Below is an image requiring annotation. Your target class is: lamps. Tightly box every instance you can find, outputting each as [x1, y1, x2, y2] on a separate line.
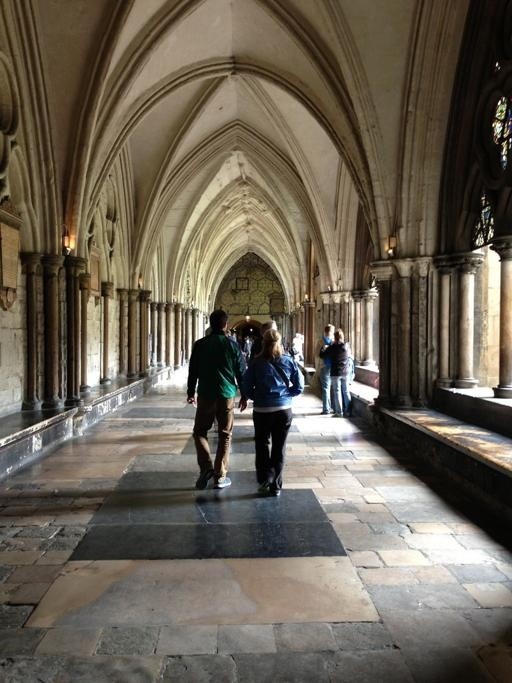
[62, 228, 76, 256]
[388, 235, 398, 250]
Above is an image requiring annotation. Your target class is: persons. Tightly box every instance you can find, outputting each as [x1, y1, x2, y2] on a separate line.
[184, 311, 355, 497]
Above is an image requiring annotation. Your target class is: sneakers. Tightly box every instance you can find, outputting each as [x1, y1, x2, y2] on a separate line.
[215, 477, 231, 488]
[196, 467, 214, 489]
